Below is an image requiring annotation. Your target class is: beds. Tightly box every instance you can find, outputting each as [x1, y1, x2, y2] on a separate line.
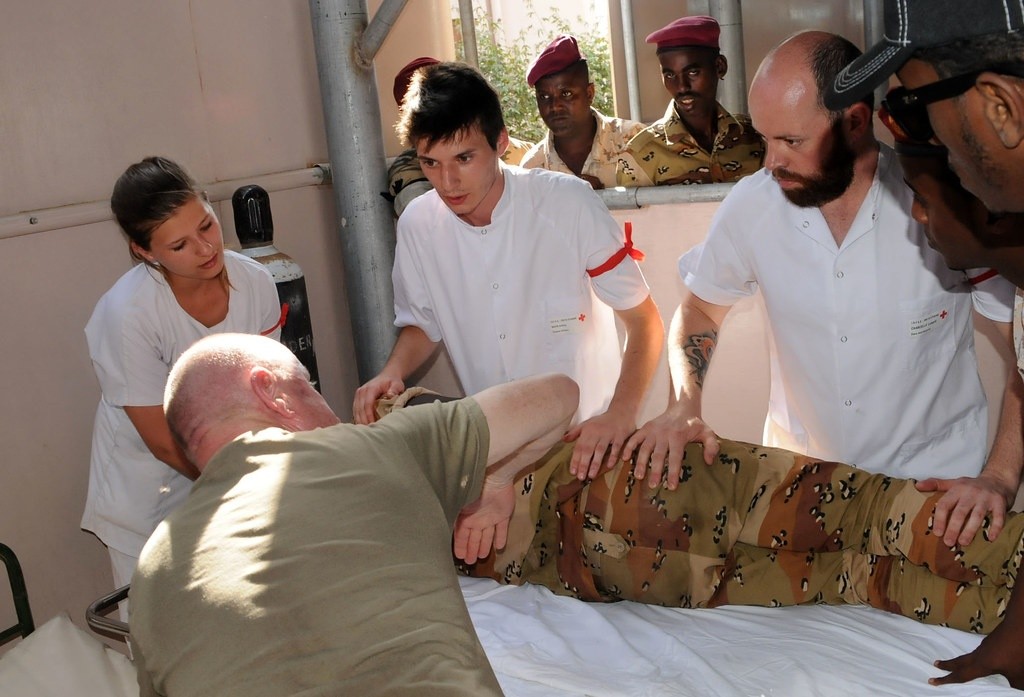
[0, 504, 1024, 697]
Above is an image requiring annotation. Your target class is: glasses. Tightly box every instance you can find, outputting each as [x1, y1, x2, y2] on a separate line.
[880, 64, 1024, 142]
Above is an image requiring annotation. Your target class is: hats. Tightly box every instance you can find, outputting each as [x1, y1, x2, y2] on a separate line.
[526, 34, 581, 88]
[393, 57, 441, 112]
[645, 15, 721, 52]
[823, 0, 1024, 111]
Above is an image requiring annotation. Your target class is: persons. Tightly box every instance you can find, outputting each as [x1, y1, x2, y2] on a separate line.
[448, 430, 1024, 636]
[519, 35, 647, 190]
[79, 157, 281, 623]
[621, 31, 988, 490]
[353, 63, 665, 481]
[822, 0, 1024, 693]
[894, 141, 1024, 547]
[615, 15, 768, 187]
[126, 332, 580, 697]
[388, 56, 536, 216]
